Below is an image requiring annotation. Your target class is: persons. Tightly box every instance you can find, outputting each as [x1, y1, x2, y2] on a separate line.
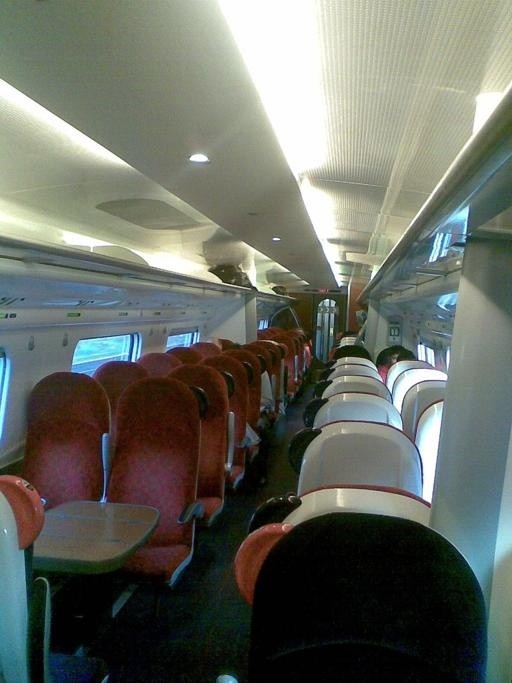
[376, 343, 405, 383]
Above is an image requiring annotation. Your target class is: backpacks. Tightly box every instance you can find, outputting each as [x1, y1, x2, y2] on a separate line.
[208, 263, 257, 290]
